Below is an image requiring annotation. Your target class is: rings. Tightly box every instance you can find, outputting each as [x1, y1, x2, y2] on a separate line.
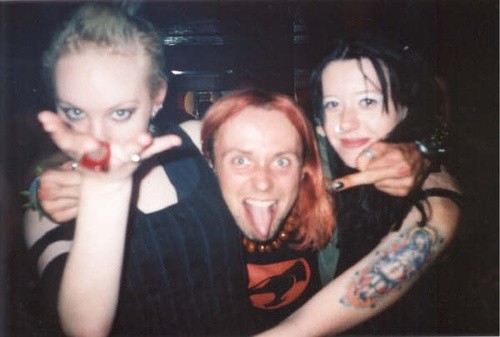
[362, 145, 379, 163]
[129, 153, 142, 165]
[80, 139, 111, 175]
[71, 160, 79, 171]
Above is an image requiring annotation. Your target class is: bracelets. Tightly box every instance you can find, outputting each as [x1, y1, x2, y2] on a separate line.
[21, 161, 64, 223]
[413, 135, 433, 159]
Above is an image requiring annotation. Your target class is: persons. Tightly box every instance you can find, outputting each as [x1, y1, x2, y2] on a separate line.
[250, 38, 474, 336]
[19, 0, 418, 337]
[27, 87, 457, 336]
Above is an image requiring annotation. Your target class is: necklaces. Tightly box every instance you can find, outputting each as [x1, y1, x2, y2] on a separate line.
[242, 200, 309, 256]
